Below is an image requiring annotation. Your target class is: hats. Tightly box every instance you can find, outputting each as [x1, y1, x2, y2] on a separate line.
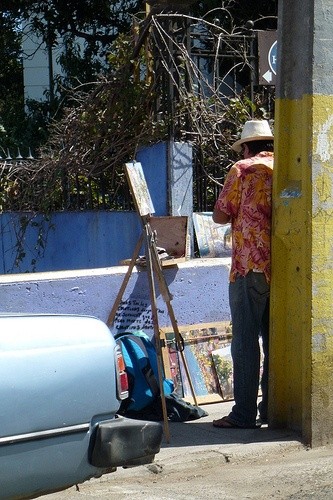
[232, 119, 275, 153]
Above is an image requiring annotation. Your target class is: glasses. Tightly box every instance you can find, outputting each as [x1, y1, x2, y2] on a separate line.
[241, 147, 246, 155]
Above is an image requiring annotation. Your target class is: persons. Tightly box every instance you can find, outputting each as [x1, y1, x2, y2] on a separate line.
[212, 120, 274, 428]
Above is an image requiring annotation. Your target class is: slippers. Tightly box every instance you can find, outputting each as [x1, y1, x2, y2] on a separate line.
[212, 415, 261, 430]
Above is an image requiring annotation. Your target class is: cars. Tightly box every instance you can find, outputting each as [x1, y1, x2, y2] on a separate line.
[0, 308, 165, 500]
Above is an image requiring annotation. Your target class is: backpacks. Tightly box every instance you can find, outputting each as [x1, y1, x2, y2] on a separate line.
[114, 331, 175, 417]
